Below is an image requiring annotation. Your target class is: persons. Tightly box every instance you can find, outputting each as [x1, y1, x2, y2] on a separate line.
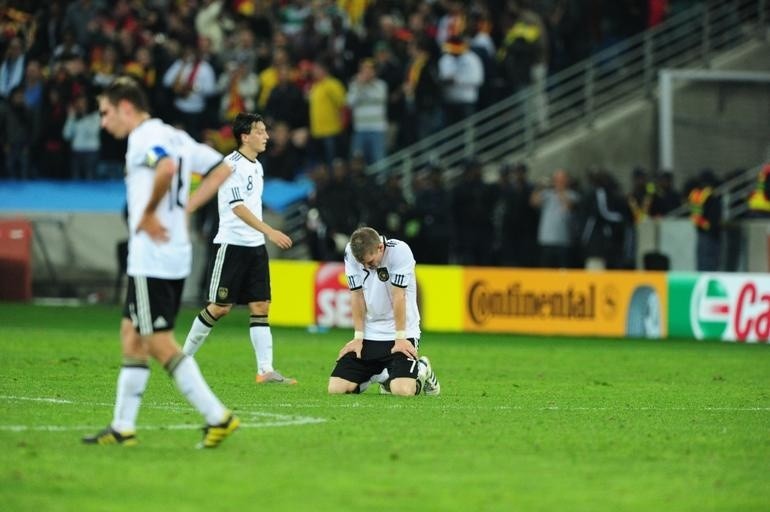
[1, 0, 545, 184]
[303, 154, 750, 272]
[82, 75, 240, 449]
[327, 227, 440, 397]
[538, 0, 749, 78]
[181, 111, 298, 383]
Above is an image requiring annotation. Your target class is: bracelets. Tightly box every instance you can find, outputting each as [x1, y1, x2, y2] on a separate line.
[395, 330, 406, 340]
[354, 330, 364, 339]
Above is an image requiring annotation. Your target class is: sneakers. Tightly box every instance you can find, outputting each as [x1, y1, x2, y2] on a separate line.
[80, 426, 141, 448]
[195, 409, 241, 451]
[378, 376, 391, 395]
[417, 355, 441, 395]
[254, 369, 300, 387]
